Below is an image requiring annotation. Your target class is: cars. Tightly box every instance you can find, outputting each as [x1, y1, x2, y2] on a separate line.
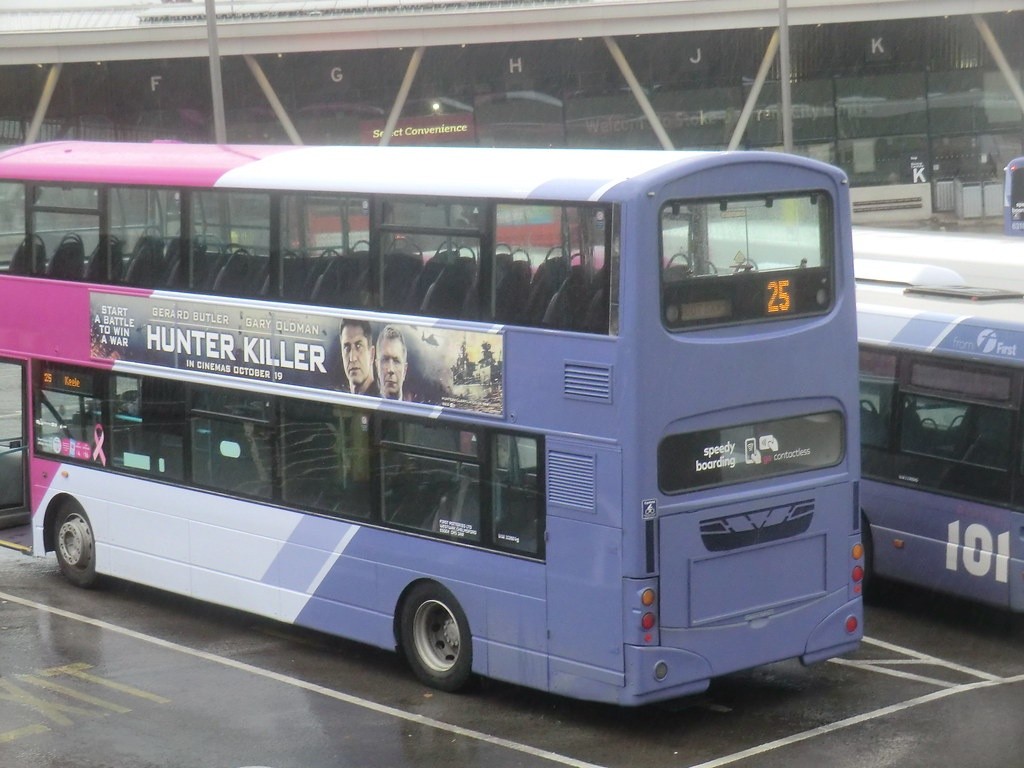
[139, 93, 561, 138]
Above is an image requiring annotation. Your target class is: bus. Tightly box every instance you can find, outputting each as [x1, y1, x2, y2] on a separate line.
[0, 139, 868, 710]
[660, 100, 837, 166]
[1003, 158, 1024, 238]
[830, 90, 989, 176]
[560, 218, 1024, 619]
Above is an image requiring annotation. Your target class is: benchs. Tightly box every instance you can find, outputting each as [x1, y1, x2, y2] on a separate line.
[662, 266, 830, 328]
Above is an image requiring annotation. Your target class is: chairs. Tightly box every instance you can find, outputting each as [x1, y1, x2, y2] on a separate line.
[86, 235, 124, 279]
[9, 234, 47, 276]
[124, 227, 719, 326]
[285, 469, 477, 532]
[860, 400, 964, 430]
[48, 232, 85, 279]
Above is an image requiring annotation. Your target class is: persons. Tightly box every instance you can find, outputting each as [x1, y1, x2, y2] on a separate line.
[367, 324, 432, 404]
[338, 319, 375, 402]
